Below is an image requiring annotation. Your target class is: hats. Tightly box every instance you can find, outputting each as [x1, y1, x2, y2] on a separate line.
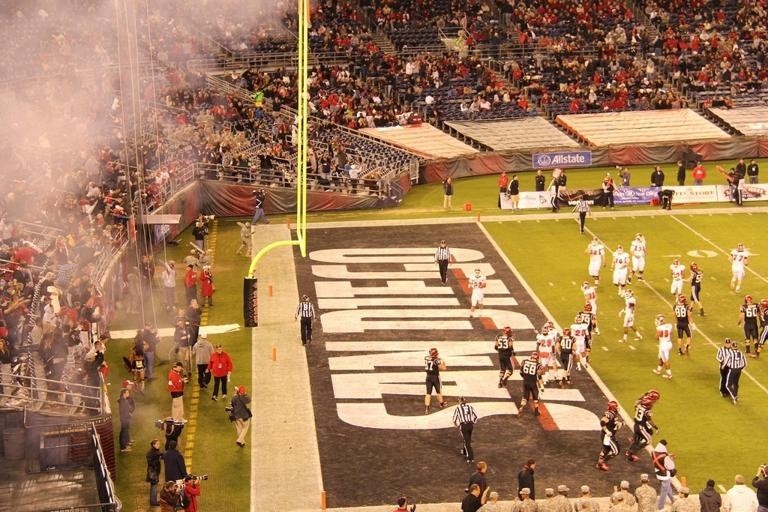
[545, 487, 554, 495]
[677, 486, 690, 494]
[489, 491, 499, 498]
[580, 485, 590, 492]
[614, 492, 623, 500]
[620, 480, 630, 488]
[519, 487, 531, 494]
[558, 484, 570, 491]
[640, 473, 649, 480]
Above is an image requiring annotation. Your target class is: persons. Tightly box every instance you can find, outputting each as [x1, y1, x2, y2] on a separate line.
[294, 294, 315, 345]
[595, 387, 767, 512]
[163, 247, 250, 449]
[572, 200, 591, 233]
[451, 396, 477, 462]
[496, 231, 768, 416]
[145, 418, 207, 512]
[118, 380, 137, 452]
[392, 497, 415, 512]
[0, 187, 159, 410]
[435, 238, 486, 321]
[2, 0, 768, 200]
[423, 348, 449, 415]
[192, 188, 272, 258]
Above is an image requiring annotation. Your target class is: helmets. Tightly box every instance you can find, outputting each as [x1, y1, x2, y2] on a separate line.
[744, 295, 753, 303]
[531, 352, 539, 361]
[641, 398, 652, 409]
[759, 299, 768, 310]
[607, 400, 619, 413]
[542, 282, 592, 336]
[736, 243, 744, 251]
[646, 389, 660, 402]
[590, 233, 699, 325]
[429, 348, 439, 357]
[502, 326, 512, 334]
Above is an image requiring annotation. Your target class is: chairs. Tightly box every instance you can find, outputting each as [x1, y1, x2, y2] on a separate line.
[0, 200, 129, 421]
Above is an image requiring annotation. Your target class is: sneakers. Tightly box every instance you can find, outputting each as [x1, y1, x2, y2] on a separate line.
[597, 461, 608, 470]
[629, 455, 641, 463]
